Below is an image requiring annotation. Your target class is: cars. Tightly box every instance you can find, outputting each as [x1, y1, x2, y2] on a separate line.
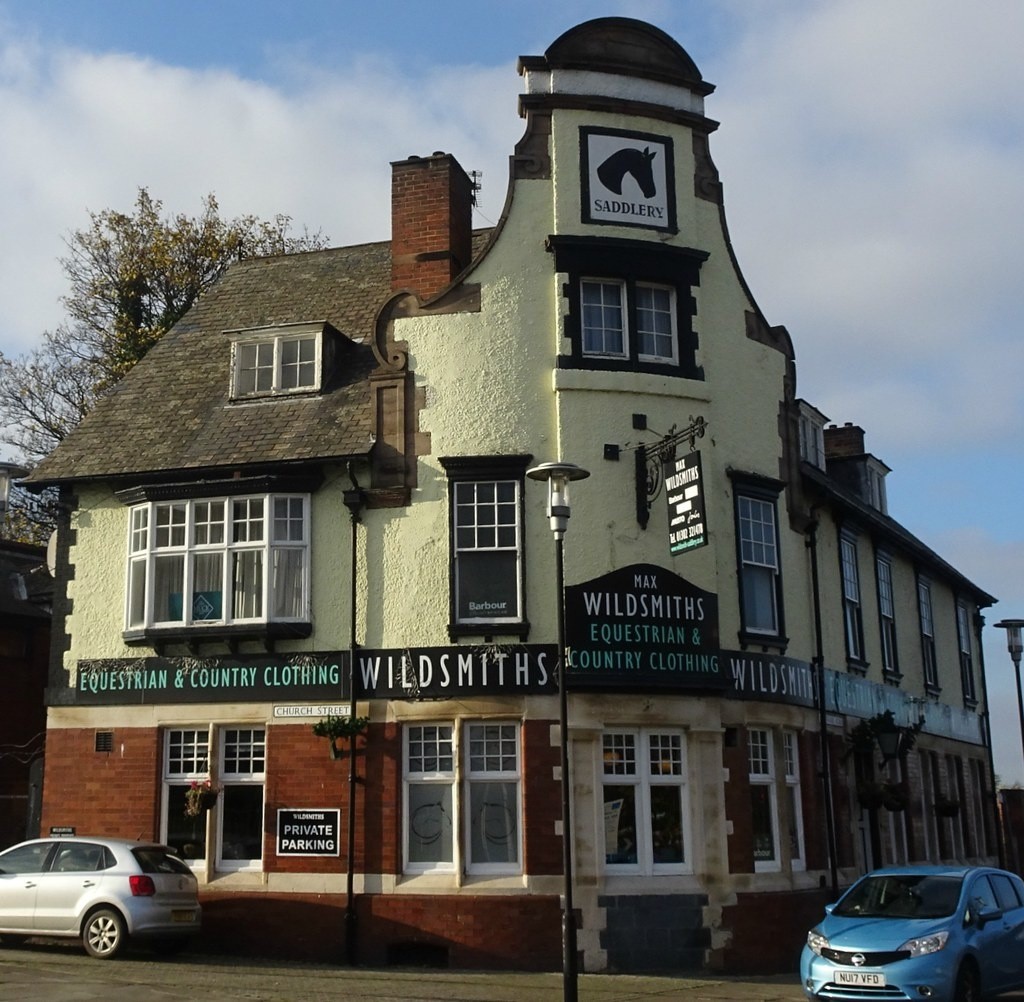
[0, 836, 203, 960]
[799, 865, 1024, 1001]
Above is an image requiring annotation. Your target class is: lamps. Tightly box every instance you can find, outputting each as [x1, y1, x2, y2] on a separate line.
[874, 723, 903, 770]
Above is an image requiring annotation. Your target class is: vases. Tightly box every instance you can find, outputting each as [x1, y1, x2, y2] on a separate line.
[941, 807, 959, 818]
[885, 799, 904, 811]
[199, 792, 215, 809]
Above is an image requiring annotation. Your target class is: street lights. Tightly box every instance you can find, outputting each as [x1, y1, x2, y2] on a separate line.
[526, 462, 591, 1002]
[993, 618, 1024, 751]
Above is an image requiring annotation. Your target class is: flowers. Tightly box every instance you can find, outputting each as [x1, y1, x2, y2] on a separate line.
[876, 781, 912, 802]
[936, 795, 962, 810]
[185, 779, 221, 817]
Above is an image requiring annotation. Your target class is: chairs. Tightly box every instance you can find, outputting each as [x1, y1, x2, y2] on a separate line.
[885, 895, 922, 916]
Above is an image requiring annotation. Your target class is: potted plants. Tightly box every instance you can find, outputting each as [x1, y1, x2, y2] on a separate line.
[311, 717, 371, 762]
